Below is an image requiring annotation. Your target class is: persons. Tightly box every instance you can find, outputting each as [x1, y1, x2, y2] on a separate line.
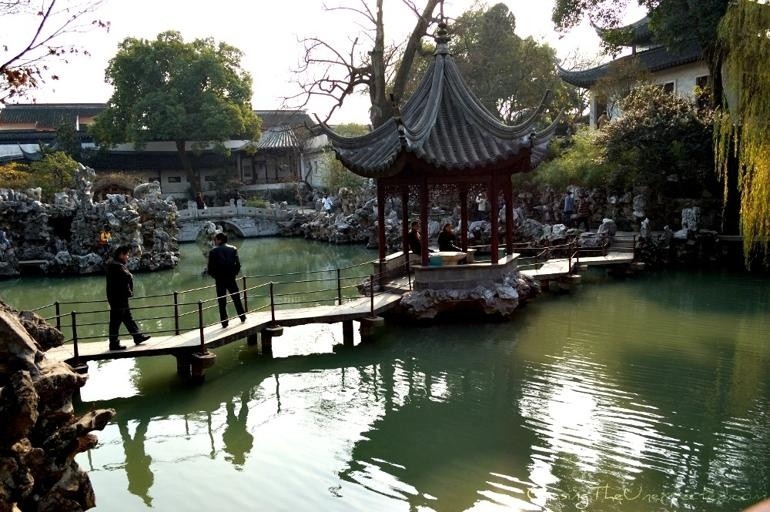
[321, 193, 335, 214]
[233, 188, 241, 206]
[115, 416, 158, 511]
[100, 225, 112, 258]
[207, 232, 247, 328]
[314, 195, 324, 212]
[409, 219, 435, 258]
[104, 245, 151, 351]
[437, 223, 462, 252]
[218, 389, 256, 472]
[467, 185, 593, 233]
[195, 191, 205, 210]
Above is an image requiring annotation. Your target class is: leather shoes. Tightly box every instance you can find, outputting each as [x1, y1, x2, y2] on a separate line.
[133, 335, 151, 345]
[110, 343, 128, 351]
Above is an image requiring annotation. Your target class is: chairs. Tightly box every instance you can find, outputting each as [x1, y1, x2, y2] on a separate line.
[429, 255, 442, 267]
